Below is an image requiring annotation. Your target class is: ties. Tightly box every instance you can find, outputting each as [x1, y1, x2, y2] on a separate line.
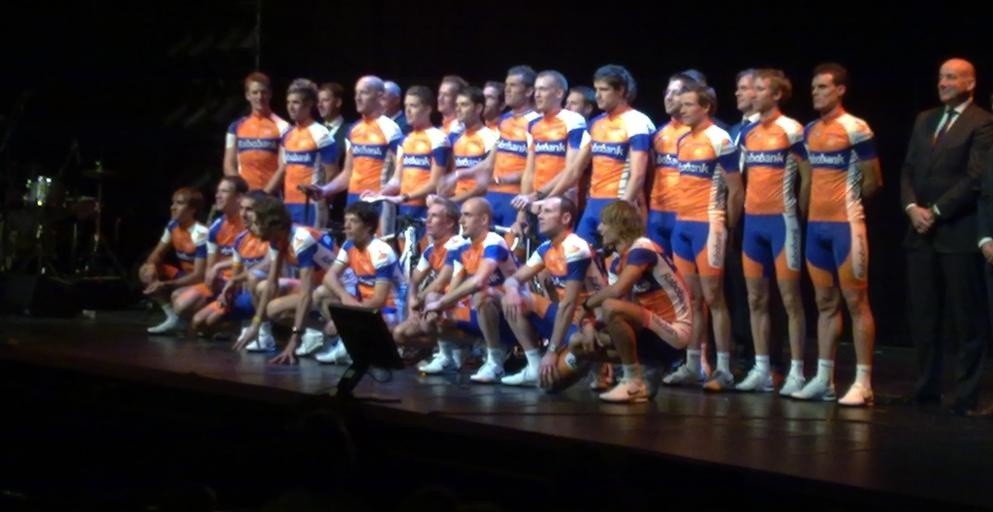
[327, 125, 333, 132]
[935, 110, 956, 143]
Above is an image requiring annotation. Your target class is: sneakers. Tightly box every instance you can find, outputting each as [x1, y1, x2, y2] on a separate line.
[599, 378, 651, 404]
[243, 335, 277, 351]
[418, 355, 459, 375]
[663, 365, 706, 387]
[778, 372, 807, 397]
[790, 378, 835, 402]
[587, 360, 619, 389]
[315, 338, 352, 363]
[145, 314, 184, 334]
[735, 369, 776, 393]
[293, 328, 326, 357]
[501, 368, 542, 389]
[837, 383, 874, 406]
[468, 361, 508, 385]
[701, 370, 737, 391]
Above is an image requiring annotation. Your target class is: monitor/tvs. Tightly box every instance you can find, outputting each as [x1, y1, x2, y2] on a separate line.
[328, 302, 405, 403]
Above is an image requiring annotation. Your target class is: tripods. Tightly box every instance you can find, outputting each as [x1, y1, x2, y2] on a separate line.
[71, 182, 133, 287]
[0, 209, 71, 286]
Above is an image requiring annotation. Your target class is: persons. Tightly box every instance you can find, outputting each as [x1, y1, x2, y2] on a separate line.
[880, 58, 992, 417]
[137, 62, 885, 408]
[962, 148, 992, 417]
